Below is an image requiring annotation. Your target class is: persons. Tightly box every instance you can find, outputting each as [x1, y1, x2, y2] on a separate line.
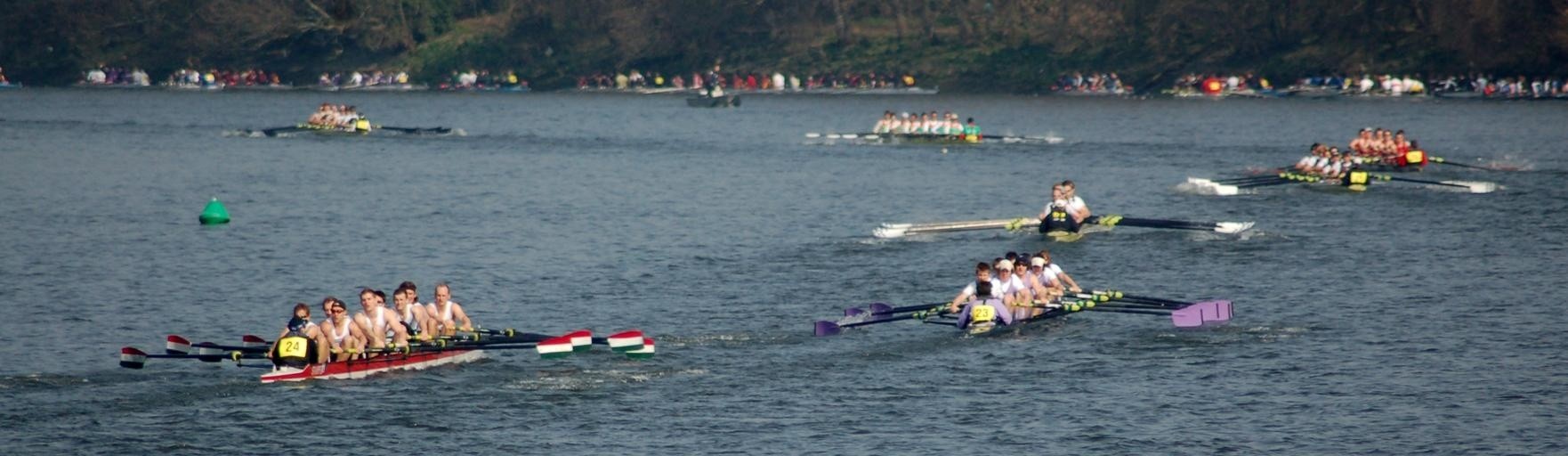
[1295, 127, 1413, 179]
[267, 281, 471, 365]
[82, 62, 280, 86]
[1038, 180, 1091, 225]
[872, 110, 982, 142]
[949, 249, 1081, 330]
[307, 70, 518, 133]
[1056, 69, 1124, 94]
[1173, 70, 1269, 92]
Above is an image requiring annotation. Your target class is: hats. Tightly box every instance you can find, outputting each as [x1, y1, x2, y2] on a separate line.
[332, 300, 346, 310]
[1031, 257, 1046, 267]
[999, 259, 1014, 271]
[1016, 256, 1028, 265]
[287, 316, 308, 331]
[1054, 199, 1067, 208]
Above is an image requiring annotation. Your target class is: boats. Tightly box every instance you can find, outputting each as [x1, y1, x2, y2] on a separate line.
[1431, 88, 1568, 100]
[1045, 224, 1085, 243]
[958, 294, 1084, 340]
[256, 337, 483, 385]
[1294, 89, 1434, 103]
[310, 126, 370, 139]
[1357, 161, 1423, 174]
[870, 133, 985, 147]
[1296, 182, 1369, 193]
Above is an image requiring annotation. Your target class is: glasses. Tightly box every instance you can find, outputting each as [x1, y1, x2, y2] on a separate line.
[331, 309, 344, 314]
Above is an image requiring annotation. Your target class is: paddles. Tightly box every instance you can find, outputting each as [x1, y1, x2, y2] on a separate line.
[1432, 157, 1518, 173]
[1082, 214, 1253, 234]
[246, 126, 322, 137]
[1026, 289, 1234, 326]
[409, 332, 646, 352]
[119, 334, 272, 370]
[446, 328, 654, 358]
[383, 327, 591, 354]
[1187, 168, 1327, 196]
[1370, 174, 1496, 194]
[814, 302, 951, 336]
[331, 337, 574, 359]
[372, 126, 451, 134]
[982, 135, 1062, 143]
[871, 218, 1041, 238]
[807, 131, 940, 140]
